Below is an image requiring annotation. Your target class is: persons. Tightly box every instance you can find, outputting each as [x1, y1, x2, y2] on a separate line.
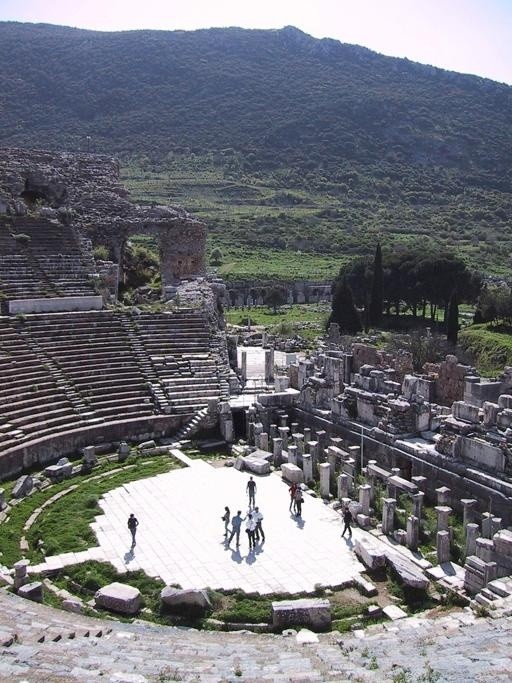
[246, 475, 258, 506]
[246, 513, 256, 549]
[340, 506, 353, 537]
[247, 506, 266, 541]
[226, 509, 247, 546]
[294, 486, 304, 517]
[223, 506, 232, 537]
[288, 482, 297, 511]
[127, 512, 140, 548]
